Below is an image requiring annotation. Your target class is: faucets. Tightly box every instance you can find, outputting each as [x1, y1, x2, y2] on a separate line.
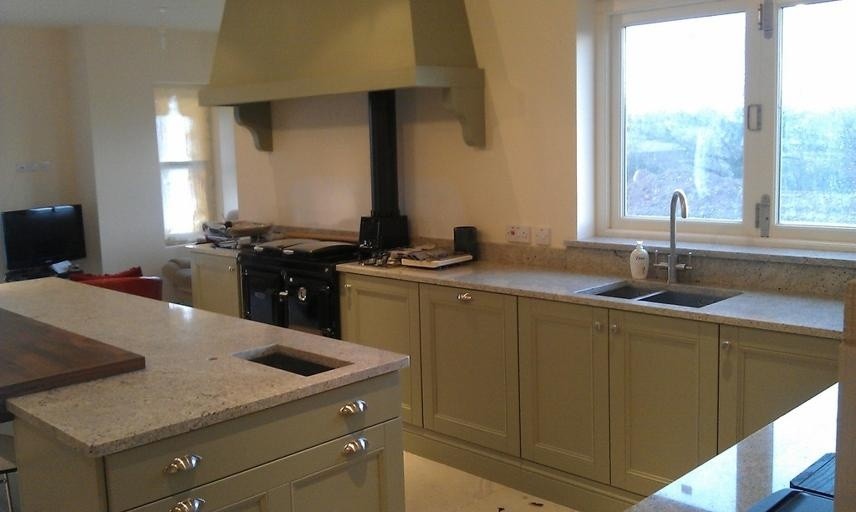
[667, 189, 687, 284]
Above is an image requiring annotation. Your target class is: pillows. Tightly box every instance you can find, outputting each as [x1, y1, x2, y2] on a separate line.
[70, 265, 142, 280]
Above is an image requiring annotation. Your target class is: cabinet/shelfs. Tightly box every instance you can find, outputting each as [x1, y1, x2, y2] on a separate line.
[520, 268, 724, 512]
[188, 254, 237, 320]
[716, 292, 838, 460]
[108, 301, 406, 510]
[344, 265, 519, 491]
[623, 385, 839, 512]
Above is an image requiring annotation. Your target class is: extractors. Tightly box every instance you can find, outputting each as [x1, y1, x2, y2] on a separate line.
[198, 0, 483, 109]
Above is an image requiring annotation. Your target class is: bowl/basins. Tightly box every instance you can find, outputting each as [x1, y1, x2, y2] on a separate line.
[227, 221, 273, 236]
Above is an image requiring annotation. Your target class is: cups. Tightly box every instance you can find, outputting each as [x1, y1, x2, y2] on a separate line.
[454, 226, 478, 259]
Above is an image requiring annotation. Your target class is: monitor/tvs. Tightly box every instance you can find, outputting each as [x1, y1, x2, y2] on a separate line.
[2, 204, 87, 269]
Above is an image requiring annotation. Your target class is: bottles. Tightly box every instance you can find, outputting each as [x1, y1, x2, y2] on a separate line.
[629, 239, 650, 281]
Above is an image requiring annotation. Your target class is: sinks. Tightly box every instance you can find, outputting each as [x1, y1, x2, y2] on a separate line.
[573, 280, 664, 299]
[637, 283, 745, 308]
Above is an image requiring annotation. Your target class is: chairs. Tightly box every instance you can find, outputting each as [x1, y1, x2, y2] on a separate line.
[69, 275, 163, 302]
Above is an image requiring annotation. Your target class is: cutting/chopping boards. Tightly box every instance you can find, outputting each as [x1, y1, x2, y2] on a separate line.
[401, 255, 473, 269]
